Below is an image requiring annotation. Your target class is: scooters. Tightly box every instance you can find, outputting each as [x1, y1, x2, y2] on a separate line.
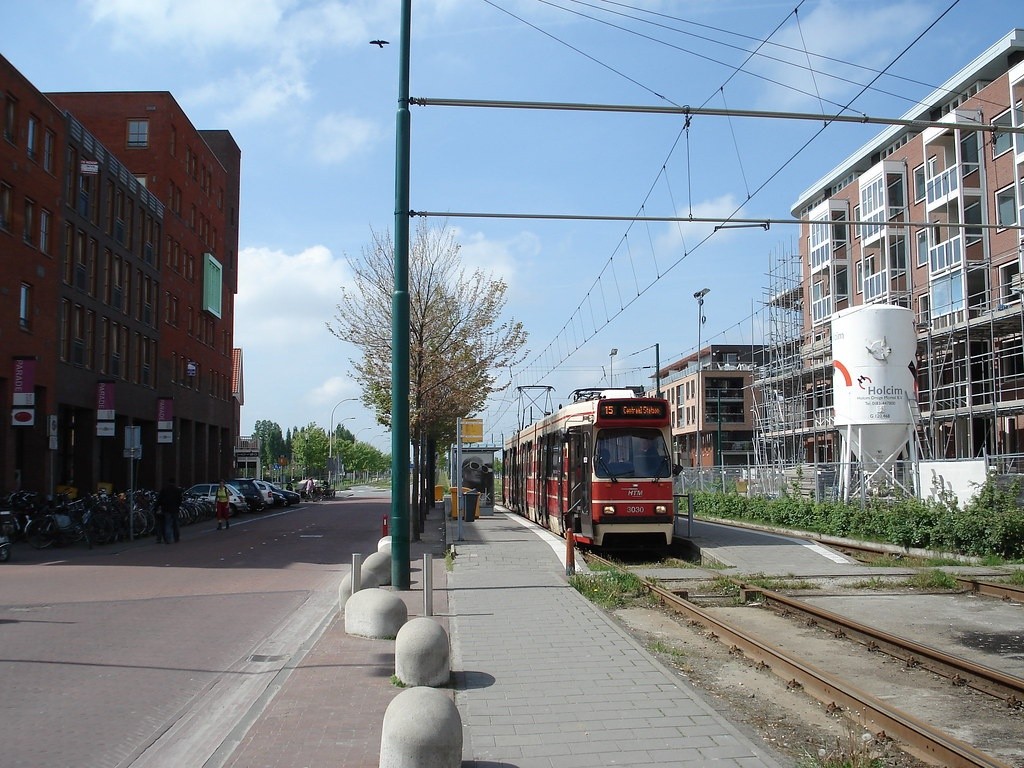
[286, 479, 294, 491]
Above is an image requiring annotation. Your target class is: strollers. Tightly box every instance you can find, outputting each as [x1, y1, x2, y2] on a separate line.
[313, 486, 325, 502]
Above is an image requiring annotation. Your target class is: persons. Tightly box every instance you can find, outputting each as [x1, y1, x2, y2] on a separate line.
[154, 476, 182, 544]
[215, 480, 230, 530]
[462, 455, 485, 490]
[286, 483, 293, 491]
[320, 481, 328, 494]
[305, 477, 317, 502]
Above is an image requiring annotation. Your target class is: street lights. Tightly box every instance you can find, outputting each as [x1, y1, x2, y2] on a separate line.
[335, 418, 355, 444]
[694, 288, 710, 490]
[356, 428, 371, 439]
[608, 349, 619, 388]
[328, 399, 358, 480]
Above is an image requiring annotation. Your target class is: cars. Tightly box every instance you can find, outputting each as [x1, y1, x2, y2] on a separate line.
[272, 492, 288, 508]
[183, 483, 247, 518]
[234, 478, 274, 512]
[227, 479, 264, 514]
[262, 481, 301, 507]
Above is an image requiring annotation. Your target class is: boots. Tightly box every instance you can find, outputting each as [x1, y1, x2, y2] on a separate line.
[217, 523, 222, 530]
[226, 521, 229, 528]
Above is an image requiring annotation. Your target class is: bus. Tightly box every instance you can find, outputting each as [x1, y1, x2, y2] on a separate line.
[502, 398, 675, 554]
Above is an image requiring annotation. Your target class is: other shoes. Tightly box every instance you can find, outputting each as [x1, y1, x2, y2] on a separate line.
[165, 539, 170, 544]
[174, 537, 179, 543]
[156, 538, 161, 543]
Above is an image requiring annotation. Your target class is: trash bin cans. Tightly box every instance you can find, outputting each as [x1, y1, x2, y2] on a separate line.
[462, 491, 480, 522]
[449, 486, 481, 520]
[435, 485, 444, 501]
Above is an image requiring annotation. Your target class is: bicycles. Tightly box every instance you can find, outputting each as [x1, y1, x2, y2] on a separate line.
[0, 487, 216, 563]
